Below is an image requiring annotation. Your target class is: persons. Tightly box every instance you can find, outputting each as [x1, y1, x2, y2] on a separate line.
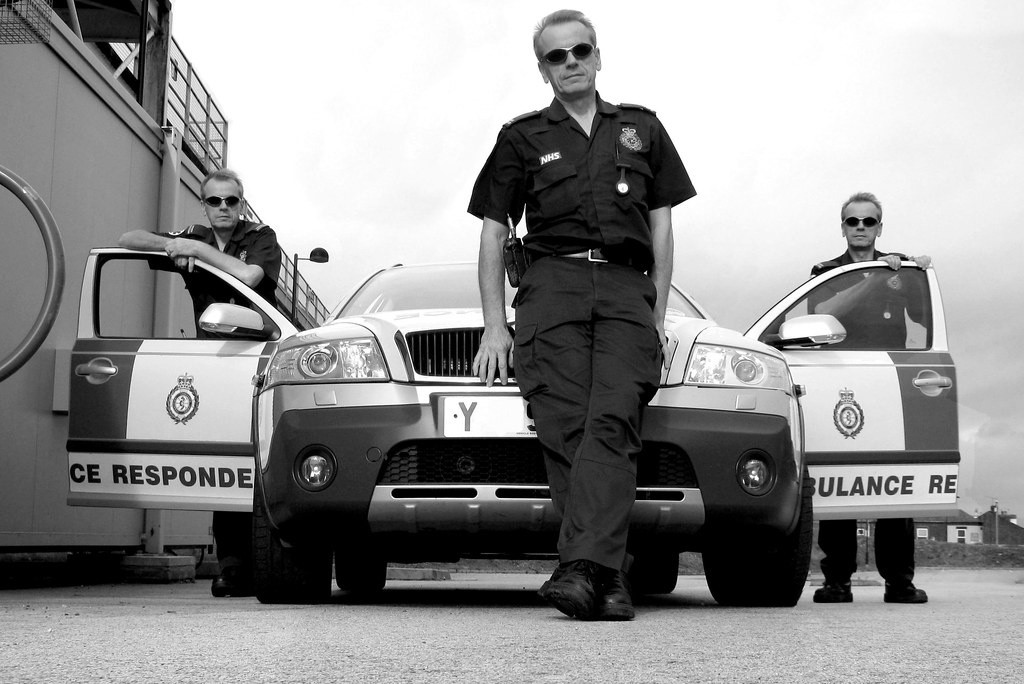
[812, 189, 935, 604]
[119, 169, 283, 598]
[466, 10, 699, 623]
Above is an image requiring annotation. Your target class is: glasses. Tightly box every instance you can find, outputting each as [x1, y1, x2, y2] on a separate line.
[840, 215, 881, 227]
[539, 41, 596, 64]
[203, 195, 243, 207]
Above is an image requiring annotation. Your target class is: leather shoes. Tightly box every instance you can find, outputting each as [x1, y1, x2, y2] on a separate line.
[537, 556, 595, 619]
[226, 571, 255, 596]
[588, 563, 635, 620]
[883, 584, 929, 604]
[811, 581, 854, 603]
[211, 563, 244, 599]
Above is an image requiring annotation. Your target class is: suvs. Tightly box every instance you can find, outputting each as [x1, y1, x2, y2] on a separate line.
[64, 247, 963, 608]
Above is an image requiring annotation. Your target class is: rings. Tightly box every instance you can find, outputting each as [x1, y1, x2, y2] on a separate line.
[169, 249, 172, 253]
